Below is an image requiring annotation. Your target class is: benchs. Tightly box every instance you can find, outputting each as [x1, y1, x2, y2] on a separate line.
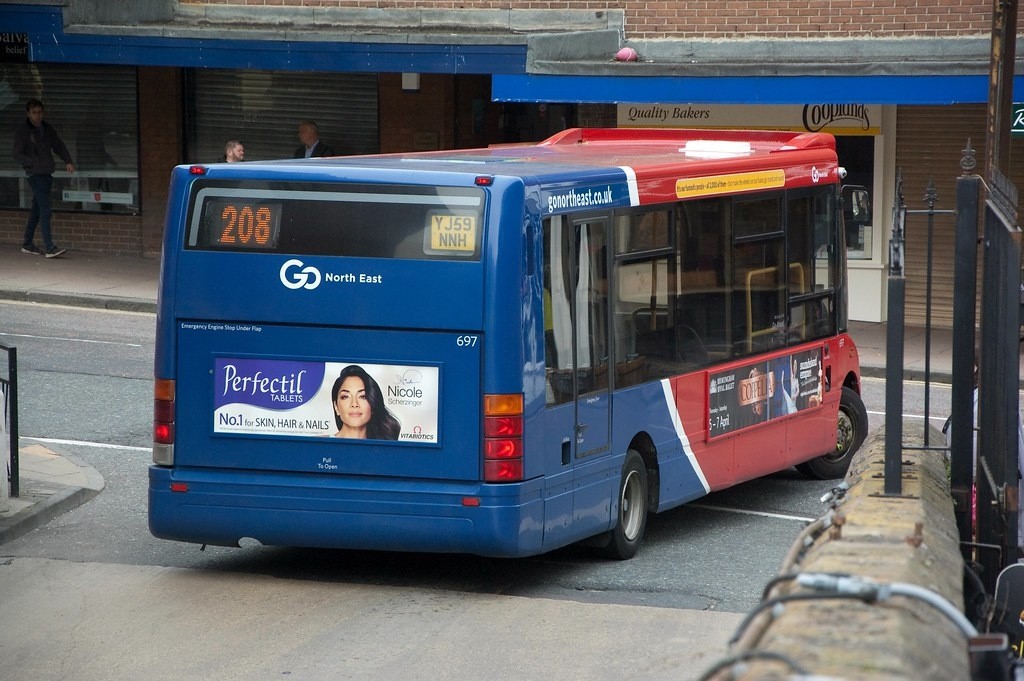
[546, 283, 827, 400]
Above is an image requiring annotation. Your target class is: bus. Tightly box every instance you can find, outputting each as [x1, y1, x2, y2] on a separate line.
[148, 128, 869, 561]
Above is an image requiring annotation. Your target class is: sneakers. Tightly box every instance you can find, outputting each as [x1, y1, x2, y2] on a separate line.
[21, 244, 43, 255]
[45, 246, 66, 258]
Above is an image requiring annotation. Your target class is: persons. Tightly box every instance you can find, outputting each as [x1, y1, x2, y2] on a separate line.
[222, 138, 245, 163]
[783, 355, 800, 414]
[331, 365, 400, 441]
[294, 121, 333, 159]
[13, 99, 73, 258]
[75, 100, 121, 210]
[855, 193, 867, 220]
